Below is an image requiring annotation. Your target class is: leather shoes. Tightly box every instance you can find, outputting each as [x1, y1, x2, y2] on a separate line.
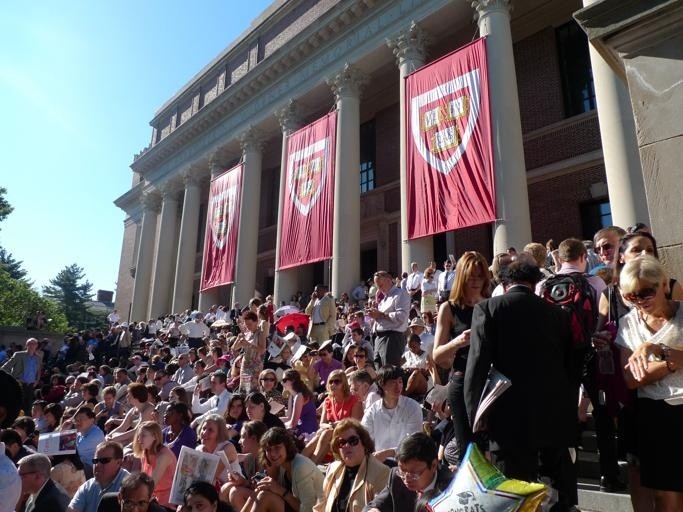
[600, 475, 622, 492]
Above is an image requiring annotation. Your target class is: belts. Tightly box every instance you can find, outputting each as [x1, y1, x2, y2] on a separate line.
[313, 322, 326, 326]
[376, 331, 404, 336]
[454, 371, 464, 375]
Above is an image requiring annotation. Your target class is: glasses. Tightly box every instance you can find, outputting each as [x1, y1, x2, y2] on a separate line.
[19, 471, 35, 476]
[337, 436, 360, 448]
[373, 272, 382, 276]
[353, 355, 366, 358]
[349, 386, 360, 392]
[468, 272, 486, 280]
[153, 375, 164, 381]
[92, 458, 113, 464]
[445, 265, 451, 268]
[329, 379, 342, 384]
[179, 356, 184, 360]
[593, 243, 612, 255]
[121, 500, 148, 510]
[260, 377, 276, 382]
[624, 283, 657, 303]
[319, 353, 328, 356]
[281, 378, 289, 383]
[422, 316, 427, 319]
[395, 466, 427, 480]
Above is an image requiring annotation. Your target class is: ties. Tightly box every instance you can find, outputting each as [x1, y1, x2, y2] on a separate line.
[444, 272, 448, 289]
[216, 398, 219, 406]
[418, 491, 424, 497]
[410, 274, 415, 289]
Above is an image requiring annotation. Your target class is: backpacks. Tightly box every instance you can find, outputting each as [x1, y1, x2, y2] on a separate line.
[541, 272, 595, 362]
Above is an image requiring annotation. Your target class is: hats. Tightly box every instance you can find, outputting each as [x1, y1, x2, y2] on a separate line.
[120, 322, 129, 328]
[408, 317, 426, 328]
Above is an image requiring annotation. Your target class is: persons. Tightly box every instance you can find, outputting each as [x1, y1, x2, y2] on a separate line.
[360, 431, 454, 512]
[305, 285, 337, 345]
[133, 420, 177, 509]
[240, 426, 324, 512]
[219, 420, 265, 511]
[233, 313, 265, 393]
[409, 315, 434, 356]
[438, 259, 454, 300]
[593, 232, 683, 354]
[162, 401, 197, 457]
[243, 393, 286, 429]
[291, 349, 321, 394]
[592, 225, 619, 287]
[203, 290, 311, 372]
[520, 242, 552, 298]
[425, 261, 439, 276]
[31, 399, 49, 431]
[105, 383, 160, 447]
[280, 370, 320, 443]
[99, 471, 170, 512]
[489, 253, 518, 297]
[62, 408, 104, 468]
[334, 278, 385, 333]
[261, 367, 286, 416]
[308, 345, 344, 389]
[68, 442, 132, 512]
[612, 251, 683, 511]
[350, 369, 378, 411]
[225, 394, 249, 440]
[0, 368, 22, 511]
[402, 333, 432, 372]
[191, 371, 231, 427]
[401, 271, 409, 290]
[182, 479, 232, 512]
[364, 364, 424, 468]
[192, 414, 246, 484]
[420, 309, 436, 333]
[17, 453, 68, 511]
[576, 267, 624, 423]
[41, 309, 210, 402]
[435, 303, 441, 313]
[96, 387, 121, 429]
[366, 270, 408, 370]
[431, 252, 495, 465]
[583, 239, 602, 272]
[463, 252, 573, 511]
[44, 403, 62, 429]
[2, 430, 37, 465]
[312, 418, 390, 512]
[429, 260, 442, 285]
[396, 276, 402, 288]
[346, 345, 377, 384]
[303, 369, 362, 469]
[535, 238, 625, 491]
[421, 269, 436, 312]
[15, 416, 36, 450]
[406, 262, 421, 304]
[1, 337, 53, 403]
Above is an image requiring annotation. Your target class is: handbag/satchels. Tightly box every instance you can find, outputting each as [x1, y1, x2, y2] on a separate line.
[598, 286, 620, 375]
